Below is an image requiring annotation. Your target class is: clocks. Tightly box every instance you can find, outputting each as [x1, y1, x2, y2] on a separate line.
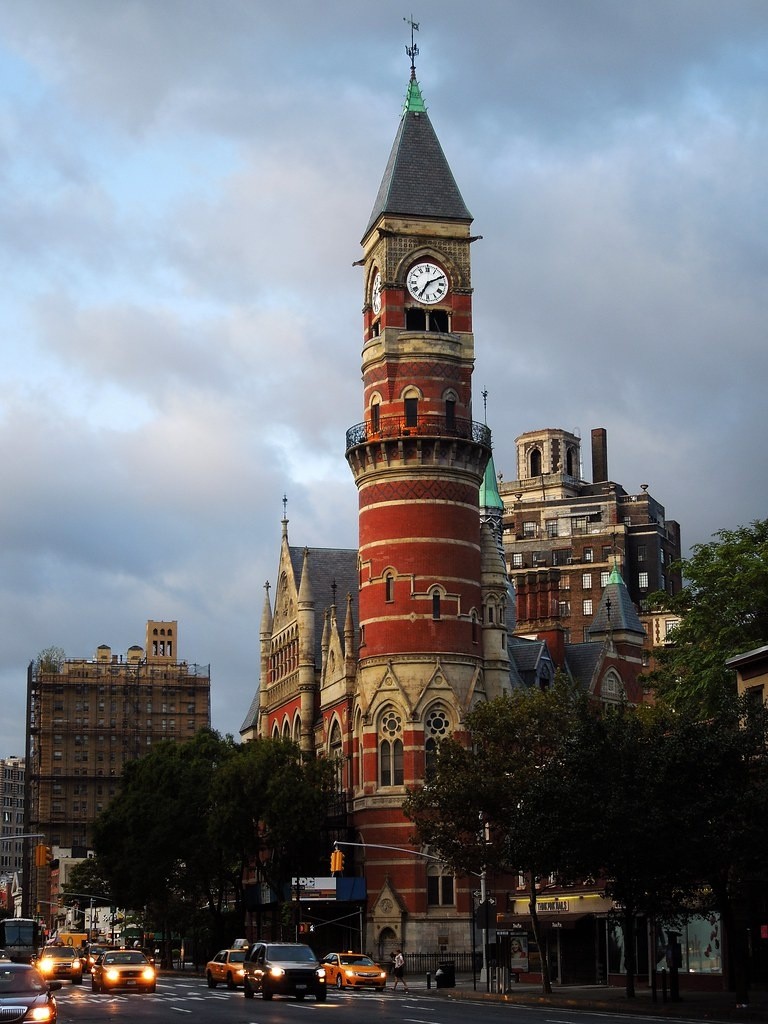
[407, 263, 449, 305]
[371, 272, 382, 315]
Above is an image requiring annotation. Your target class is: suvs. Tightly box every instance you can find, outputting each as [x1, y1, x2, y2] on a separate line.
[244, 939, 327, 1003]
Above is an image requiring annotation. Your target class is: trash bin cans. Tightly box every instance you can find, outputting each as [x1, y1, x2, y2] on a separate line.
[437, 960, 455, 988]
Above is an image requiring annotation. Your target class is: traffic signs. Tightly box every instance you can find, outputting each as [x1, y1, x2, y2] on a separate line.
[479, 897, 497, 907]
[472, 889, 491, 899]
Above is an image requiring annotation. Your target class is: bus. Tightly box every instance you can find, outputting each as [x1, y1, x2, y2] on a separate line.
[0, 918, 40, 965]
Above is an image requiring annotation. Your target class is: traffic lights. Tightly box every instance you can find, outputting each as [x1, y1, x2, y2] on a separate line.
[301, 923, 308, 933]
[40, 845, 51, 866]
[335, 852, 346, 872]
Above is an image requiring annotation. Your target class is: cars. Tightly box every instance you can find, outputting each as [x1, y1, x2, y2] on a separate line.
[32, 936, 87, 986]
[91, 946, 156, 993]
[0, 963, 63, 1024]
[0, 949, 12, 963]
[320, 950, 386, 992]
[85, 937, 117, 973]
[204, 939, 252, 991]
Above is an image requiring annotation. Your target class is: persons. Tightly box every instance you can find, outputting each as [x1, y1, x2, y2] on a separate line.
[511, 938, 527, 958]
[609, 920, 627, 973]
[392, 950, 408, 991]
